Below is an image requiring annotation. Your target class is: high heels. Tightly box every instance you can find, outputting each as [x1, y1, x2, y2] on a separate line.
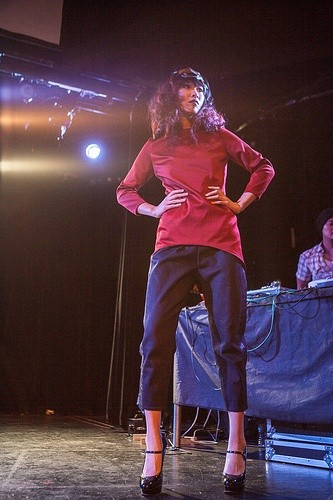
[223, 445, 246, 489]
[139, 438, 168, 494]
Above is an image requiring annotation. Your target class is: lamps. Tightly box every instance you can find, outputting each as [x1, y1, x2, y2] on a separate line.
[85, 142, 110, 166]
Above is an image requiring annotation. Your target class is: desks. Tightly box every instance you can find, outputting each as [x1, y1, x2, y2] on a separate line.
[173, 285, 333, 449]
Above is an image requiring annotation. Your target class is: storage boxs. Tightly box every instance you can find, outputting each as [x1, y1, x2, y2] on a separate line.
[265, 417, 333, 445]
[265, 440, 333, 470]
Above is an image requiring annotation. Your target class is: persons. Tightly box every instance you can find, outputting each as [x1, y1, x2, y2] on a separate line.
[115, 67, 276, 498]
[294, 216, 333, 291]
